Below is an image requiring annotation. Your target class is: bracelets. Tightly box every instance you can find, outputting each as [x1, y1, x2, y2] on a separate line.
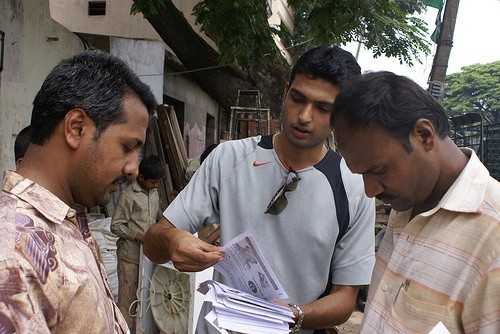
[288, 303, 304, 334]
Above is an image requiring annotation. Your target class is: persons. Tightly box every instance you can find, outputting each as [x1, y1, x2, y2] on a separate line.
[0, 52, 222, 334]
[329, 72, 500, 334]
[143, 47, 375, 334]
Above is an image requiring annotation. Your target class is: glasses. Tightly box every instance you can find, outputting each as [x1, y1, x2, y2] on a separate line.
[264, 167, 300, 216]
